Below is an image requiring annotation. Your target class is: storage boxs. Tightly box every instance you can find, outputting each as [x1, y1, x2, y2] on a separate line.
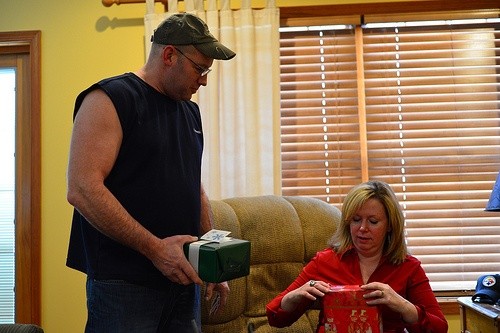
[323, 285, 384, 333]
[184, 236, 251, 283]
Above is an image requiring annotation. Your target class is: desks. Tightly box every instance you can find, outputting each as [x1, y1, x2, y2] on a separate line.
[456, 297, 500, 332]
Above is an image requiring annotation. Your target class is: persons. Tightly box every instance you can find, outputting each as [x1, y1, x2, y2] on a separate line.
[66, 14, 236, 333]
[265, 181, 449, 333]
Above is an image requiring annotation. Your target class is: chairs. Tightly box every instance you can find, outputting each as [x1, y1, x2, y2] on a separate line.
[199, 195, 344, 333]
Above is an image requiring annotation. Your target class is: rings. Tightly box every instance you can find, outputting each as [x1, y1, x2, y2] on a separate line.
[310, 280, 316, 287]
[382, 290, 384, 297]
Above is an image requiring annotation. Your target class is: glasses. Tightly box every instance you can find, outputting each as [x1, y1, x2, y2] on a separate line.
[162, 45, 212, 77]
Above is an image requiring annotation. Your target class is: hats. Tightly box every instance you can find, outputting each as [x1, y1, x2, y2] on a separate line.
[150, 12, 236, 60]
[471, 274, 500, 305]
